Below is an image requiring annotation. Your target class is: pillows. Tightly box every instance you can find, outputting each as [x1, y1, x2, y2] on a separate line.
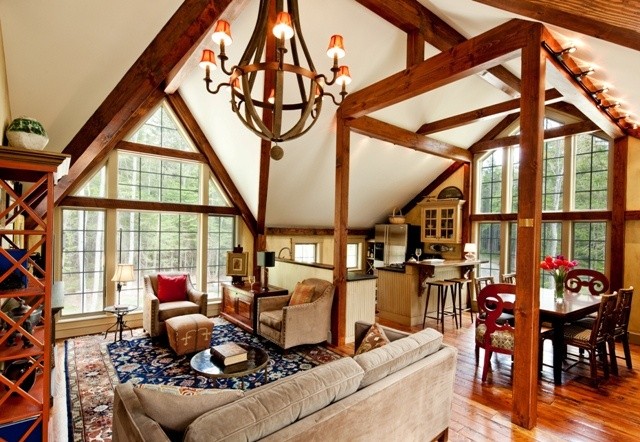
[157, 274, 187, 304]
[288, 282, 315, 307]
[354, 324, 391, 356]
[133, 385, 243, 432]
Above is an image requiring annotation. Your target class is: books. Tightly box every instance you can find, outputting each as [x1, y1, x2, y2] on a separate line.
[1, 249, 28, 288]
[210, 342, 247, 366]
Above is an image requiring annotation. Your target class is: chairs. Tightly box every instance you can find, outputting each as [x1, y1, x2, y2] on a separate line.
[573, 286, 634, 377]
[474, 277, 514, 325]
[564, 269, 610, 295]
[501, 273, 516, 284]
[554, 291, 618, 388]
[475, 284, 516, 385]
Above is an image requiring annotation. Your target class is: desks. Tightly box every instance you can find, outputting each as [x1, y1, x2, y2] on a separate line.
[403, 259, 489, 327]
[485, 288, 601, 389]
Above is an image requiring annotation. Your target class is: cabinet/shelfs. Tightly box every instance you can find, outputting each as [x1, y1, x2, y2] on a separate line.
[376, 269, 413, 326]
[417, 197, 466, 244]
[345, 278, 377, 345]
[373, 223, 420, 276]
[220, 282, 288, 333]
[1, 145, 71, 442]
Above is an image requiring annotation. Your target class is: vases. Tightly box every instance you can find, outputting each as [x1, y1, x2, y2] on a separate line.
[554, 279, 565, 303]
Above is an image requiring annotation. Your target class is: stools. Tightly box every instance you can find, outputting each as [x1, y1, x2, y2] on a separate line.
[422, 281, 458, 333]
[444, 278, 474, 329]
[164, 314, 214, 355]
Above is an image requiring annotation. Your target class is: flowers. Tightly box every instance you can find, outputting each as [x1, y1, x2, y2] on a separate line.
[540, 254, 578, 298]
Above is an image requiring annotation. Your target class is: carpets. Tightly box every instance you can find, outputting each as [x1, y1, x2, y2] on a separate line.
[65, 316, 345, 441]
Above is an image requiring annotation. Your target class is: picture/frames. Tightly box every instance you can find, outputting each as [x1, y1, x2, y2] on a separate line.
[225, 251, 248, 276]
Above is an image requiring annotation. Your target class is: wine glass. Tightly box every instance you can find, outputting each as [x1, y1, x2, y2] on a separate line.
[415, 249, 422, 265]
[248, 275, 256, 293]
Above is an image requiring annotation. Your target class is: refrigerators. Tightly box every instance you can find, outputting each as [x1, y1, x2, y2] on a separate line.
[374, 224, 408, 267]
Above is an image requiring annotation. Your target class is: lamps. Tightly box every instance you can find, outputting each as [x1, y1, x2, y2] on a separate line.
[110, 264, 136, 309]
[198, 0, 350, 160]
[257, 250, 275, 290]
[464, 243, 477, 260]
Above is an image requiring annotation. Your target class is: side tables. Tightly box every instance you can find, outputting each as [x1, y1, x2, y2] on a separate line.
[103, 306, 138, 344]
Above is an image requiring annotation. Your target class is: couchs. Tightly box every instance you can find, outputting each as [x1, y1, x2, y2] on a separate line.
[112, 322, 456, 442]
[257, 278, 336, 355]
[142, 274, 207, 347]
